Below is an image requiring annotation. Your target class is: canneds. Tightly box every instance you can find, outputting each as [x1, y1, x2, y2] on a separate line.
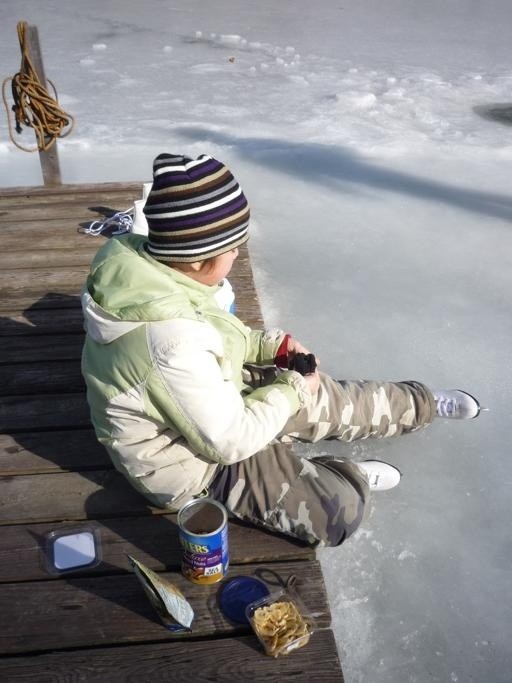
[174, 496, 232, 586]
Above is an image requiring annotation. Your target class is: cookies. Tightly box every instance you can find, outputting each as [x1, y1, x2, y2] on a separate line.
[251, 601, 310, 657]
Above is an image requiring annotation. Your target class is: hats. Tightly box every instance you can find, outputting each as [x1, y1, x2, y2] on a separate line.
[143, 153, 250, 263]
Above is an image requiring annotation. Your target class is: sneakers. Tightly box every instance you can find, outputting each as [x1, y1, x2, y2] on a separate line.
[433, 389, 480, 419]
[358, 460, 401, 491]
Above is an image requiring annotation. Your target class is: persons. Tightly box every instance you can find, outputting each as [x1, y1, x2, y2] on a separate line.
[79, 150, 482, 548]
